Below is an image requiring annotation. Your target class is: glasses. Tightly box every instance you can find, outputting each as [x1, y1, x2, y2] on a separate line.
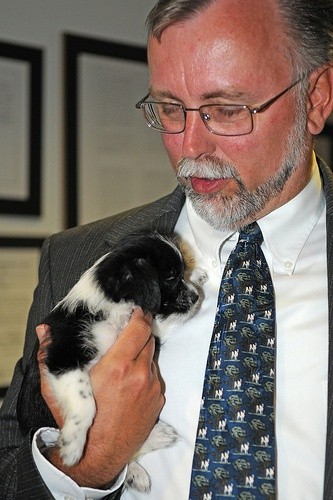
[135, 73, 309, 136]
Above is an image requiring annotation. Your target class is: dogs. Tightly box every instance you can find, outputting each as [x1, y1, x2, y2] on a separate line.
[36, 227, 209, 496]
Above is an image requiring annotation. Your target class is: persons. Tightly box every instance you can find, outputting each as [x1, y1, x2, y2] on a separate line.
[0, 1, 333, 497]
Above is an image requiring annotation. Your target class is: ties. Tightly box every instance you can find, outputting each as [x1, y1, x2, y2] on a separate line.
[188, 220, 277, 500]
